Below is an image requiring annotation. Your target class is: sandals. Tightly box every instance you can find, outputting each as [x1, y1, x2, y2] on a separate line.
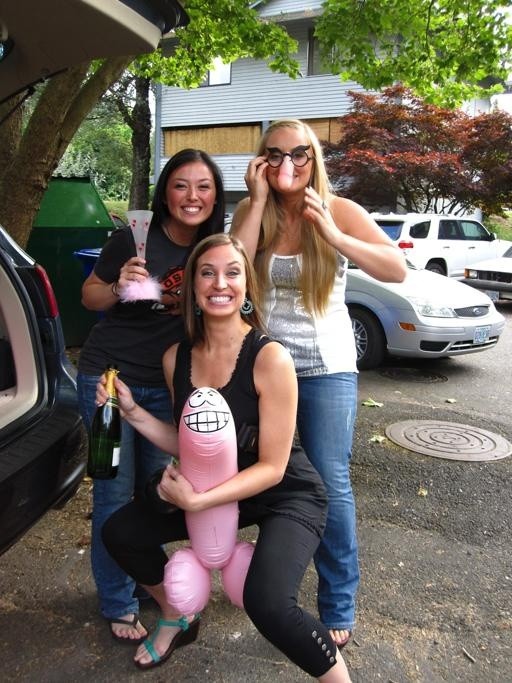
[327, 630, 352, 651]
[110, 615, 149, 644]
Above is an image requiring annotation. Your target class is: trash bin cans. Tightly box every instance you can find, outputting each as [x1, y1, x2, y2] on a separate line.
[73, 248, 106, 321]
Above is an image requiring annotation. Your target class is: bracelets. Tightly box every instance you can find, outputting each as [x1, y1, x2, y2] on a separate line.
[111, 282, 119, 297]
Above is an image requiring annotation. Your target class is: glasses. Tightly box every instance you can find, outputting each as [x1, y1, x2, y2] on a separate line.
[265, 150, 313, 166]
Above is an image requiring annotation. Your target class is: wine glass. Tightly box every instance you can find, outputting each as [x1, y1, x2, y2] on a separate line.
[126, 208, 155, 266]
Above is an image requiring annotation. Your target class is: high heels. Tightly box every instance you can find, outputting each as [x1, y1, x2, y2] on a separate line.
[134, 612, 200, 669]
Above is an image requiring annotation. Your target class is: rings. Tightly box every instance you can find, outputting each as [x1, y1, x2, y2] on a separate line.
[322, 200, 328, 210]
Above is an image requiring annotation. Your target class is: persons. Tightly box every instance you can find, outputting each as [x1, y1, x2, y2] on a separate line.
[76, 147, 225, 643]
[231, 118, 408, 651]
[100, 231, 355, 683]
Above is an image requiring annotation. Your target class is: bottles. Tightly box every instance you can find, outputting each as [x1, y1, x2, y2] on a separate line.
[88, 363, 122, 478]
